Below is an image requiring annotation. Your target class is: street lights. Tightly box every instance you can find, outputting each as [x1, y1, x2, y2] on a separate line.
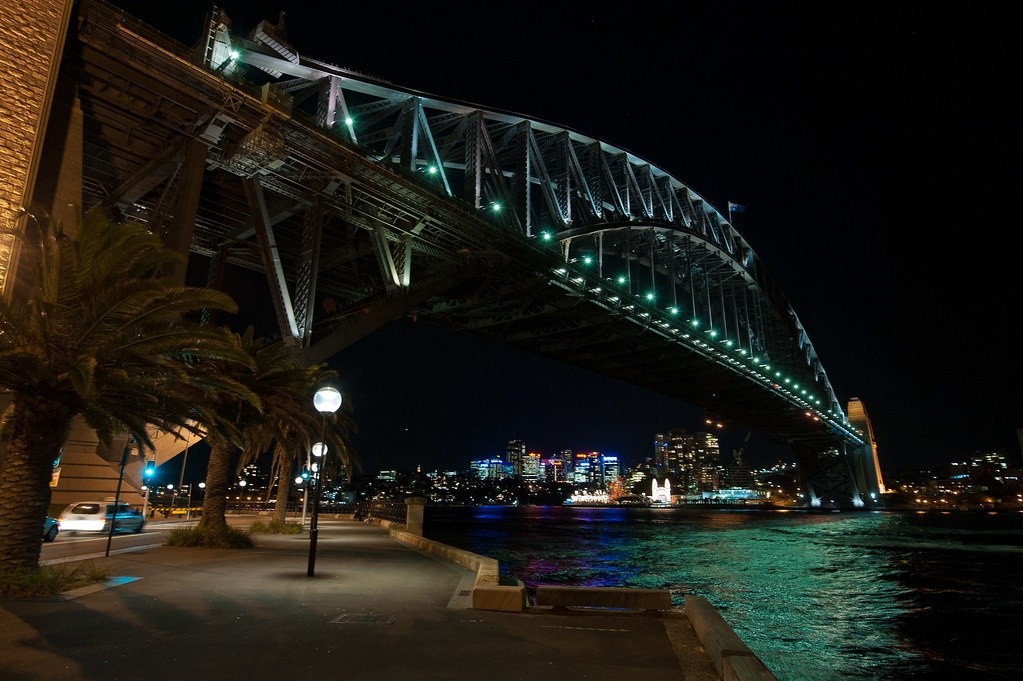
[307, 387, 342, 576]
[195, 482, 206, 518]
[309, 441, 328, 538]
[238, 481, 246, 514]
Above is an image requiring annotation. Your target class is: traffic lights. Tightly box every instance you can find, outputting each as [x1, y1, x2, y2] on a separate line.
[145, 461, 155, 478]
[303, 464, 310, 479]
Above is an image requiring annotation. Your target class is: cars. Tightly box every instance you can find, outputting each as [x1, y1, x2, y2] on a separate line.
[58, 500, 144, 533]
[43, 515, 60, 542]
